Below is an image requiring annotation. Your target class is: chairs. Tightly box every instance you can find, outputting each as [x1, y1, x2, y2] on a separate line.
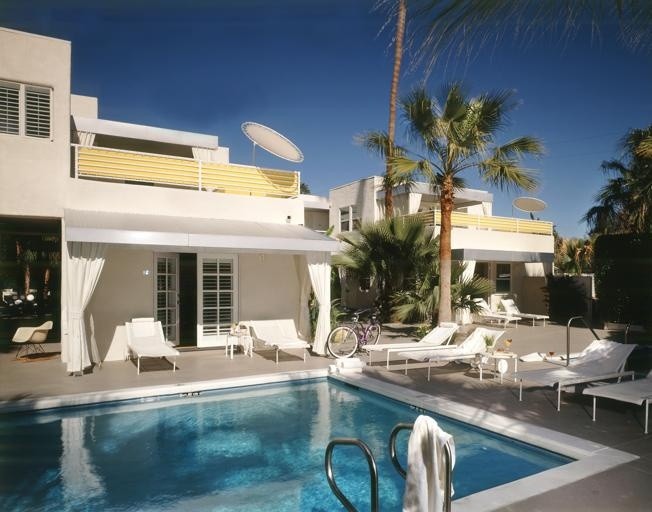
[250, 319, 311, 365]
[11, 320, 54, 359]
[400, 327, 506, 382]
[500, 299, 549, 327]
[361, 322, 459, 376]
[507, 339, 639, 412]
[583, 369, 651, 435]
[125, 317, 180, 375]
[473, 297, 522, 329]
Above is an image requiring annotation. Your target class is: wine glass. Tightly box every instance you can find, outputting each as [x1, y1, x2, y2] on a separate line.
[549, 352, 554, 359]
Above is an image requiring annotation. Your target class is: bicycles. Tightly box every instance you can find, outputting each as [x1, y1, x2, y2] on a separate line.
[327, 309, 381, 358]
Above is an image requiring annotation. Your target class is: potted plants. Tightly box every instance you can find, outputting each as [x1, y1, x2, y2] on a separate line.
[484, 336, 494, 354]
[451, 274, 497, 326]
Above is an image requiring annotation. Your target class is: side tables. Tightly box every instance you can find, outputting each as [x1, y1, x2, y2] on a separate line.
[479, 352, 518, 385]
[226, 333, 253, 360]
